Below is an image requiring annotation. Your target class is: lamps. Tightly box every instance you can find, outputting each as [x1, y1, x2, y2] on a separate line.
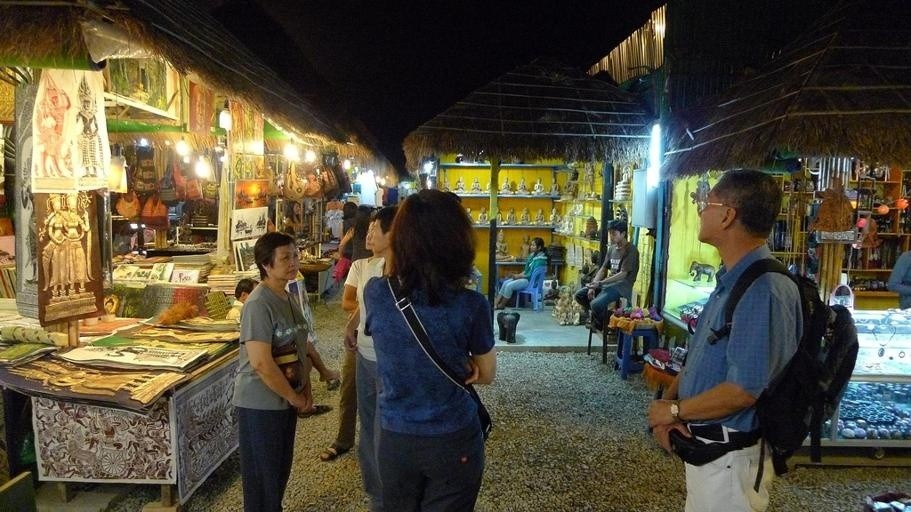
[219, 100, 231, 130]
[305, 145, 319, 163]
[176, 137, 188, 155]
[286, 138, 298, 160]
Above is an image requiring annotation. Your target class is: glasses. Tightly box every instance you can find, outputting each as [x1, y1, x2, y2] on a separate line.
[697, 198, 734, 214]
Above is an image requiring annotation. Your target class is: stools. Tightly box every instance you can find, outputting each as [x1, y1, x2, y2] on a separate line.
[613, 330, 660, 380]
[588, 297, 630, 364]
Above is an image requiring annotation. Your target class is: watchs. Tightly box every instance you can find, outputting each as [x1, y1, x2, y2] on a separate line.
[598, 281, 602, 288]
[670, 399, 689, 423]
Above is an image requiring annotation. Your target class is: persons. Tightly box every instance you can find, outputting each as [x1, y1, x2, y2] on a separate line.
[338, 206, 374, 261]
[476, 207, 489, 224]
[40, 194, 67, 299]
[456, 177, 465, 193]
[117, 191, 138, 217]
[226, 278, 341, 418]
[284, 200, 304, 239]
[547, 178, 560, 194]
[344, 206, 398, 511]
[444, 177, 449, 191]
[364, 189, 495, 512]
[574, 219, 639, 333]
[495, 229, 513, 262]
[520, 235, 530, 258]
[342, 202, 357, 276]
[888, 251, 911, 310]
[71, 73, 104, 181]
[142, 192, 167, 217]
[231, 232, 313, 512]
[471, 178, 481, 193]
[496, 207, 504, 225]
[515, 177, 530, 194]
[646, 169, 802, 512]
[535, 208, 547, 225]
[549, 208, 561, 225]
[35, 73, 70, 179]
[67, 194, 95, 298]
[505, 208, 517, 224]
[519, 208, 532, 225]
[544, 278, 561, 301]
[494, 238, 551, 310]
[501, 178, 514, 194]
[181, 177, 216, 244]
[532, 177, 545, 194]
[319, 217, 376, 461]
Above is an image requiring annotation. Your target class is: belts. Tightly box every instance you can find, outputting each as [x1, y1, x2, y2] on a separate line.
[683, 423, 749, 442]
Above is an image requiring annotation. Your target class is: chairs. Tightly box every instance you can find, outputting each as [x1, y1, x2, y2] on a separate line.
[515, 267, 548, 311]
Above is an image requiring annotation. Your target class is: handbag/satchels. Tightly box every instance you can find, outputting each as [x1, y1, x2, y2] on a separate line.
[116, 191, 140, 218]
[203, 157, 219, 198]
[159, 149, 180, 207]
[140, 191, 168, 226]
[388, 274, 491, 437]
[272, 291, 304, 390]
[283, 155, 352, 200]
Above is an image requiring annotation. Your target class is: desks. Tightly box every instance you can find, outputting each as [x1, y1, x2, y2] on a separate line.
[299, 261, 331, 309]
[643, 355, 688, 434]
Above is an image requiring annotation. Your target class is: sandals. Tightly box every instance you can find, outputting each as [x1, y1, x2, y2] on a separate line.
[320, 443, 351, 461]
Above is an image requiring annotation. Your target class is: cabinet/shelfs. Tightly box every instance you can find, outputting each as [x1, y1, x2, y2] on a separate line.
[552, 162, 613, 296]
[798, 312, 910, 455]
[437, 162, 566, 296]
[769, 168, 911, 295]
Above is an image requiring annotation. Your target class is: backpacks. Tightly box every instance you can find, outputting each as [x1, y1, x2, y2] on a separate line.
[688, 258, 859, 493]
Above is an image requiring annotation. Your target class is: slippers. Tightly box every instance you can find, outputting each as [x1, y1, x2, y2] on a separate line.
[328, 370, 342, 390]
[298, 404, 333, 418]
